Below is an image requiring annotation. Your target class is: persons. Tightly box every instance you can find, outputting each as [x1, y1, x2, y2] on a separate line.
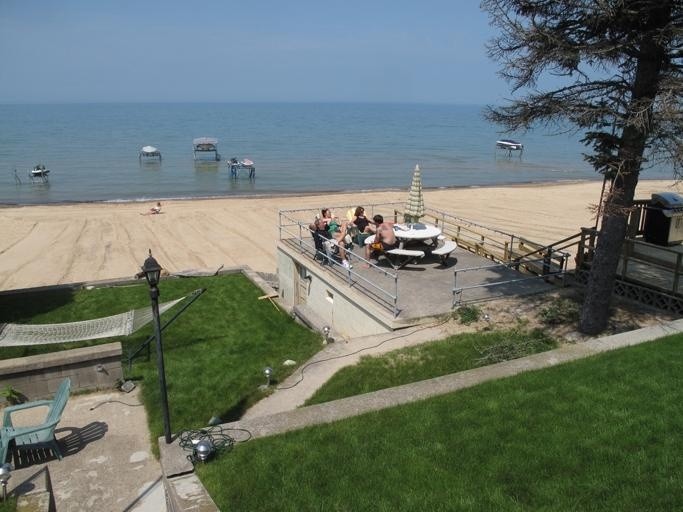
[138, 202, 161, 217]
[309, 207, 399, 270]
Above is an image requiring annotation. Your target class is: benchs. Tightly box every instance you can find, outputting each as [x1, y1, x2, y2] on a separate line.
[364, 234, 376, 244]
[432, 241, 457, 255]
[436, 235, 446, 240]
[386, 249, 425, 258]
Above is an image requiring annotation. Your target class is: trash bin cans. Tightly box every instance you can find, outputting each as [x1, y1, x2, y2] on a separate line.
[643, 192, 683, 246]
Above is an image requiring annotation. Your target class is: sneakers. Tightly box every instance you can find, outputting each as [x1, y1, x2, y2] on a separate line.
[342, 259, 352, 269]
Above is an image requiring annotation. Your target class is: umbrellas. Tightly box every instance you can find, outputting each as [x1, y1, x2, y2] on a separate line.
[403, 166, 425, 223]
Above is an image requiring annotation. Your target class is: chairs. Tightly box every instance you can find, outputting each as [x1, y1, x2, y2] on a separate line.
[0, 376, 71, 468]
[346, 207, 371, 247]
[313, 252, 326, 266]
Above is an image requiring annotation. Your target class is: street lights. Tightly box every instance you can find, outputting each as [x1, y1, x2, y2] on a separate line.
[140, 248, 172, 443]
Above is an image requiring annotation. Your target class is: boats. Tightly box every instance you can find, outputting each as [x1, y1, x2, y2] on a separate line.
[32, 169, 50, 174]
[496, 139, 521, 150]
